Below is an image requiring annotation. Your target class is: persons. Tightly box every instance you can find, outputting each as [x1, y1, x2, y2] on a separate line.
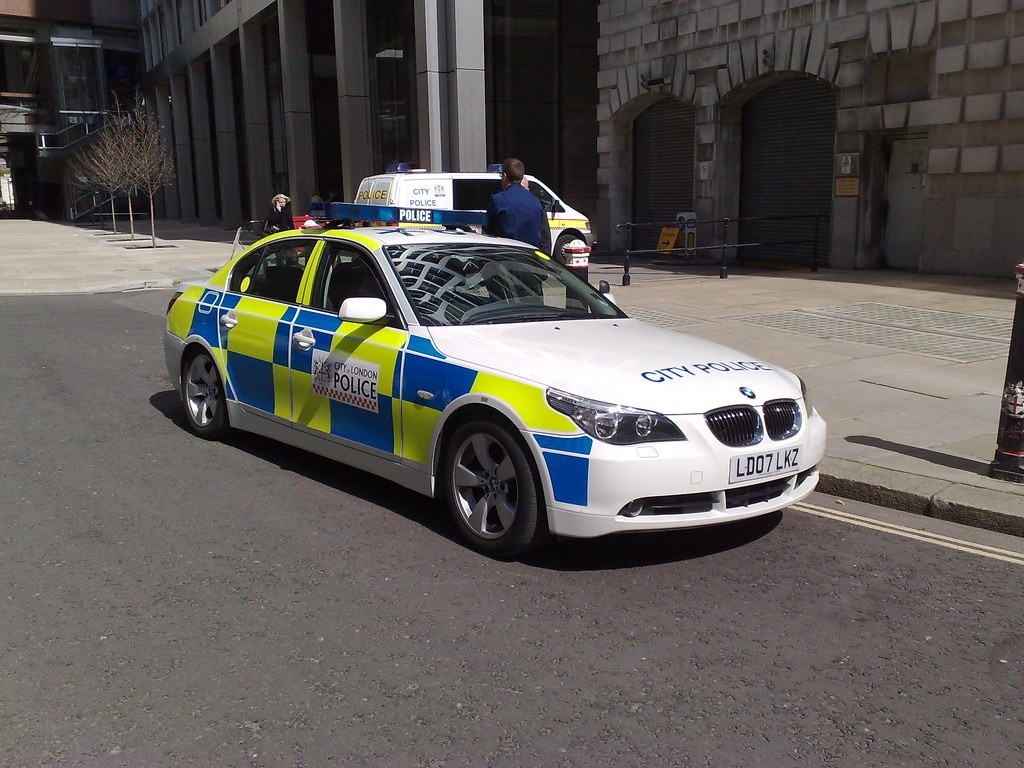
[268, 194, 295, 268]
[485, 158, 544, 250]
[310, 187, 326, 216]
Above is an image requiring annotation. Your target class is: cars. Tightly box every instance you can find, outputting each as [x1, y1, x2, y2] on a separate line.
[162, 201, 828, 564]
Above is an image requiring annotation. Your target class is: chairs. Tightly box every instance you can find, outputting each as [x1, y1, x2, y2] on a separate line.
[266, 247, 305, 296]
[424, 262, 486, 305]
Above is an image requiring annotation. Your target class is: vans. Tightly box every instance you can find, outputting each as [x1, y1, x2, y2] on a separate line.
[354, 162, 594, 267]
